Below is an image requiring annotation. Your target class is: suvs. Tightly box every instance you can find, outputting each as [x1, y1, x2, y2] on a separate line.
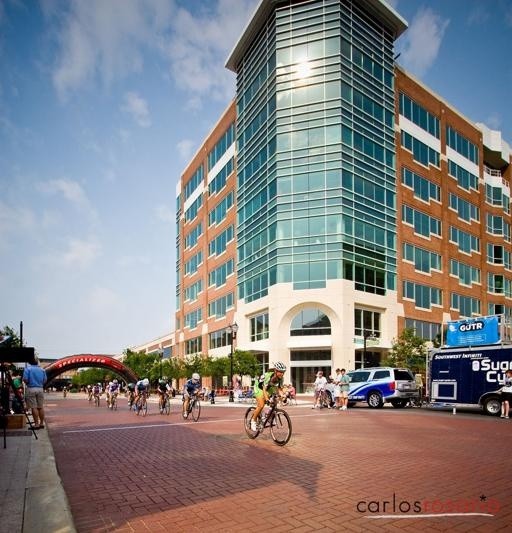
[320, 367, 420, 408]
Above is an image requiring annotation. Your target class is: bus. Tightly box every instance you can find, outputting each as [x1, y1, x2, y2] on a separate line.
[424, 342, 512, 417]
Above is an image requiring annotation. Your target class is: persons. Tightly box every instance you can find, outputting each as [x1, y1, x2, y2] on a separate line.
[0, 361, 31, 416]
[311, 370, 332, 409]
[337, 368, 350, 410]
[248, 361, 291, 432]
[326, 372, 332, 383]
[331, 367, 342, 409]
[23, 355, 49, 431]
[61, 371, 216, 418]
[230, 374, 296, 406]
[498, 368, 512, 419]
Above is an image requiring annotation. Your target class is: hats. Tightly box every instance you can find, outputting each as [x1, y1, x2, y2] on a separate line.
[335, 369, 345, 371]
[316, 371, 323, 376]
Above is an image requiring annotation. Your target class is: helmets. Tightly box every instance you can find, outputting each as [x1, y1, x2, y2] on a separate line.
[192, 373, 200, 380]
[162, 376, 168, 380]
[143, 378, 149, 386]
[273, 362, 286, 372]
[108, 379, 118, 385]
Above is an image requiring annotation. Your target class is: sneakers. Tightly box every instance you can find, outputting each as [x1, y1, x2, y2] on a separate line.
[160, 406, 163, 412]
[328, 405, 347, 410]
[106, 397, 112, 408]
[501, 415, 509, 419]
[312, 404, 323, 408]
[128, 401, 138, 410]
[250, 418, 257, 432]
[28, 425, 45, 430]
[184, 411, 187, 418]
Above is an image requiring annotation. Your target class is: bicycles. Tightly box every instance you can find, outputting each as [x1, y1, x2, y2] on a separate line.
[317, 389, 325, 408]
[86, 391, 150, 417]
[181, 391, 205, 422]
[158, 390, 172, 416]
[243, 391, 292, 447]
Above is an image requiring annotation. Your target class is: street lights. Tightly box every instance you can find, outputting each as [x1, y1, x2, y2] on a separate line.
[225, 321, 238, 402]
[157, 349, 164, 381]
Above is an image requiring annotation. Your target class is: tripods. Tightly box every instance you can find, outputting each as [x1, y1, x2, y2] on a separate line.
[0, 365, 38, 448]
